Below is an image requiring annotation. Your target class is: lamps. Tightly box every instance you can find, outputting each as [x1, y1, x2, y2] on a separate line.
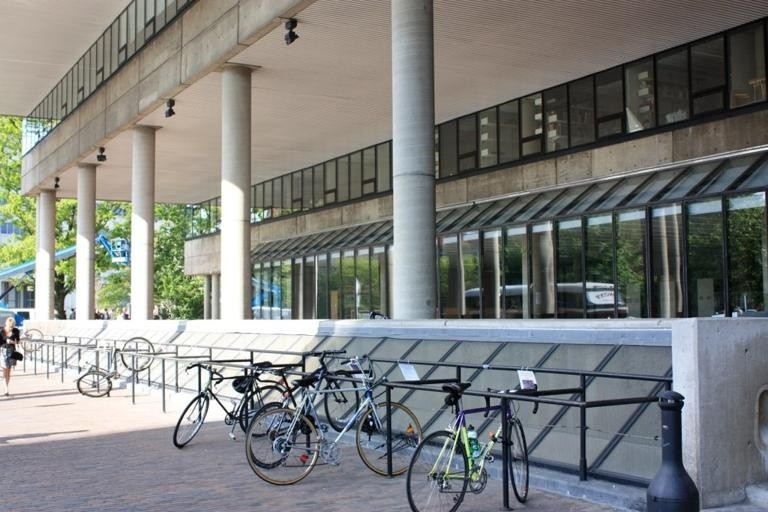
[165, 98, 175, 119]
[96, 147, 106, 162]
[53, 178, 59, 190]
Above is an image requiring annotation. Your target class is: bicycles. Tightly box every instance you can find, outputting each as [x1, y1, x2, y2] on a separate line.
[173, 349, 423, 485]
[18, 329, 44, 353]
[407, 381, 539, 512]
[72, 337, 155, 397]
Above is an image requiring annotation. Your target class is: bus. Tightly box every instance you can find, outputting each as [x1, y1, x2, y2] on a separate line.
[464, 282, 629, 319]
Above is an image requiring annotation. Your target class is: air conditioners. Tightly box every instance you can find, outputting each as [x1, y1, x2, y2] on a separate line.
[283, 18, 299, 45]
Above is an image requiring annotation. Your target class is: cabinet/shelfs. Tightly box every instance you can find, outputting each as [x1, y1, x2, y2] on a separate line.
[480, 109, 518, 166]
[546, 104, 609, 151]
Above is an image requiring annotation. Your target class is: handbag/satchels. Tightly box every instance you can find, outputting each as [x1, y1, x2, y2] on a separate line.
[11, 352, 23, 360]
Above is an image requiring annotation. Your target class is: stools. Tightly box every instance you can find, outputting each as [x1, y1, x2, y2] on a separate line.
[747, 77, 766, 102]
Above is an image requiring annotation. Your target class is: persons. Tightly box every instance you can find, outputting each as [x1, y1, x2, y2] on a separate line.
[62, 307, 129, 320]
[0, 317, 20, 397]
